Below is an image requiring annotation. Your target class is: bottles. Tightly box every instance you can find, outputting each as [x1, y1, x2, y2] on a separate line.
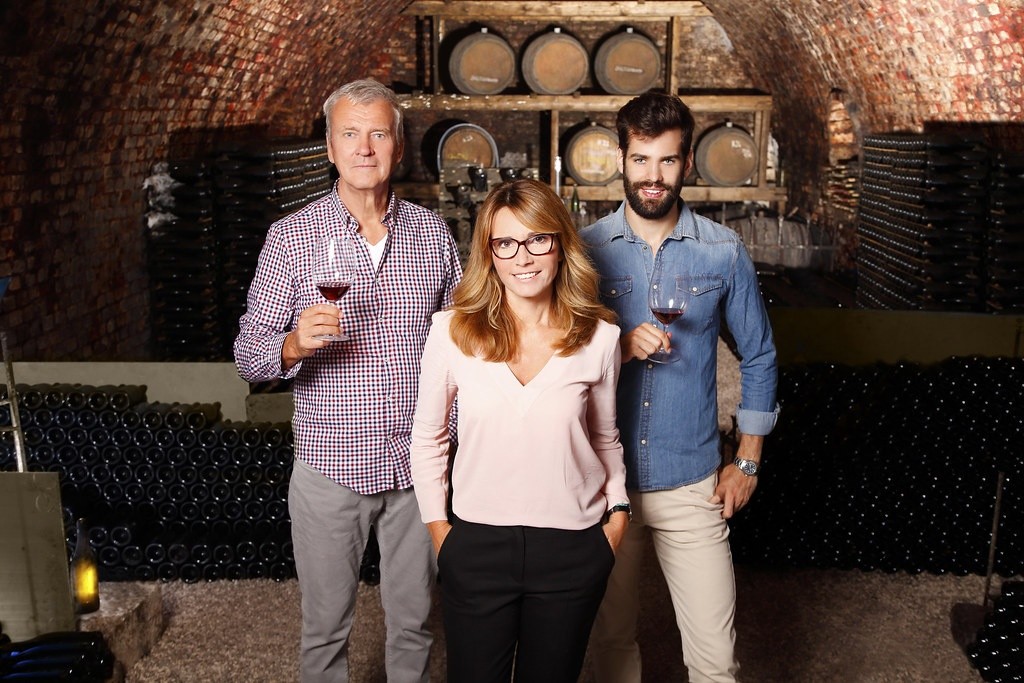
[0, 630, 116, 683]
[149, 140, 334, 364]
[745, 130, 1024, 314]
[719, 353, 1024, 683]
[1, 378, 381, 587]
[571, 183, 579, 212]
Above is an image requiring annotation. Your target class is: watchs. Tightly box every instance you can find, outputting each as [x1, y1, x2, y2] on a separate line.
[607, 502, 633, 526]
[733, 456, 759, 477]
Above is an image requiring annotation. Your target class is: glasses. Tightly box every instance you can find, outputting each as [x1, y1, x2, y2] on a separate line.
[488, 232, 559, 259]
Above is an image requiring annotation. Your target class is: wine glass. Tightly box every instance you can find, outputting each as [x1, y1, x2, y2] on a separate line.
[313, 237, 355, 342]
[646, 269, 688, 364]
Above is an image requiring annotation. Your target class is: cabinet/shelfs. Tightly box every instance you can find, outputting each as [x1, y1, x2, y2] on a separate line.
[387, 16, 786, 200]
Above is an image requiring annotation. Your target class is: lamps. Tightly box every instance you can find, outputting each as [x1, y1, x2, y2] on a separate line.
[77, 560, 100, 613]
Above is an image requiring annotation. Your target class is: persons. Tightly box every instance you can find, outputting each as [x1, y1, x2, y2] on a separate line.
[233, 78, 465, 683]
[574, 91, 777, 683]
[409, 179, 633, 683]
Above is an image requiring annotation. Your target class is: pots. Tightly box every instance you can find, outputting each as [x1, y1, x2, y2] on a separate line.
[448, 27, 515, 95]
[521, 27, 589, 95]
[695, 122, 758, 187]
[594, 27, 661, 95]
[565, 122, 623, 187]
[437, 123, 499, 173]
